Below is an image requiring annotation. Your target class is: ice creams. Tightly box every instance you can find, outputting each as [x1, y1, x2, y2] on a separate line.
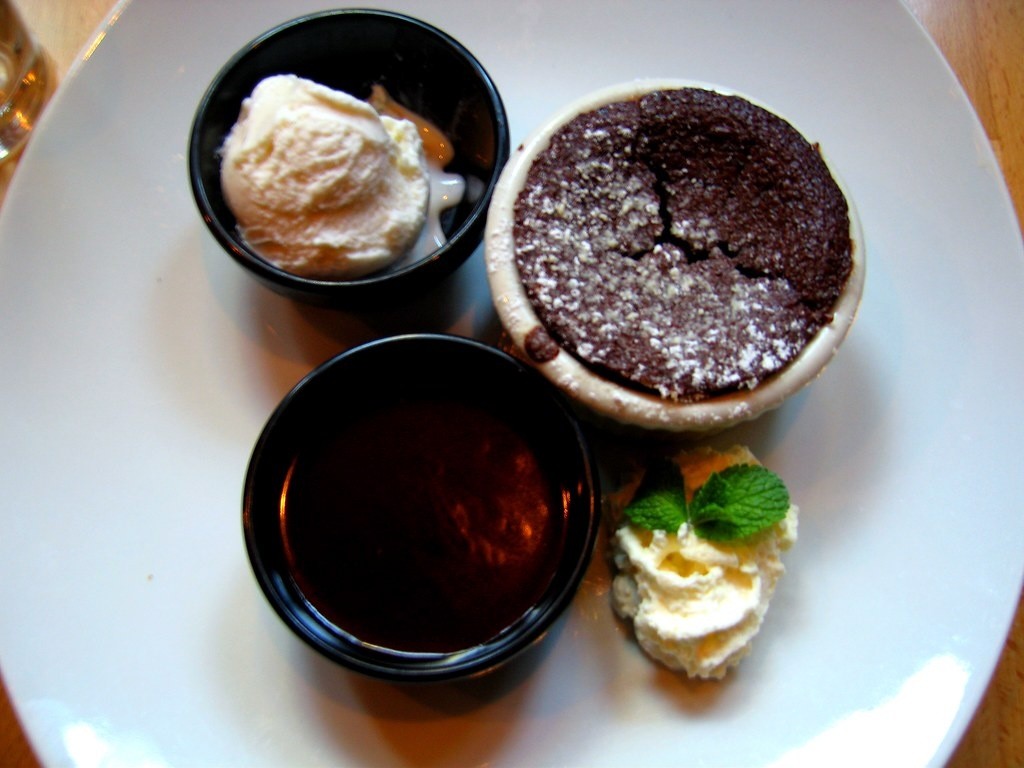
[219, 70, 466, 283]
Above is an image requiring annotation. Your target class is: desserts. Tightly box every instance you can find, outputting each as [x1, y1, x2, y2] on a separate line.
[520, 90, 852, 677]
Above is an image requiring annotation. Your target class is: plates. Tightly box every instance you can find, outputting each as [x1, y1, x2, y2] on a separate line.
[0, 0, 1023, 768]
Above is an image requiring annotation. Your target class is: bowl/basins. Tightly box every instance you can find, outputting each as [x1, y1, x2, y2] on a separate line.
[482, 76, 871, 438]
[238, 329, 603, 678]
[185, 3, 514, 305]
